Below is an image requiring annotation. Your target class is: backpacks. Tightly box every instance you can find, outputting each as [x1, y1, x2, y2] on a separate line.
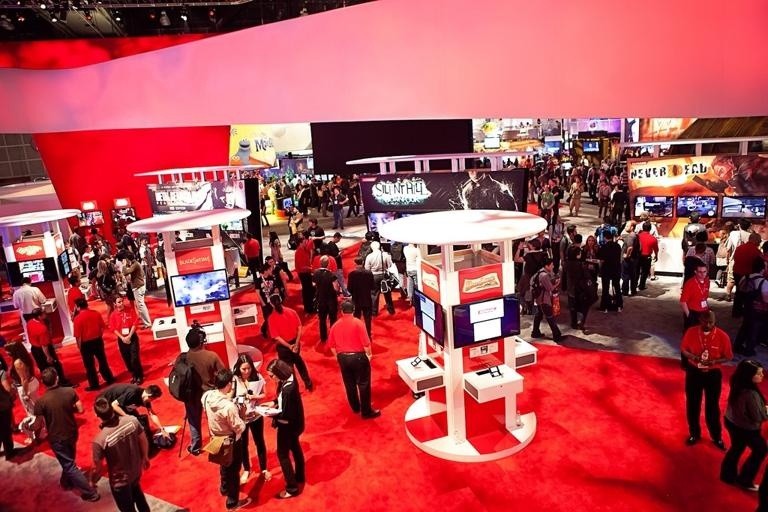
[169, 352, 193, 401]
[530, 270, 548, 299]
[733, 273, 764, 311]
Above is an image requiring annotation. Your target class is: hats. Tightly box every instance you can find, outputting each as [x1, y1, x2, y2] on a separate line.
[342, 302, 355, 310]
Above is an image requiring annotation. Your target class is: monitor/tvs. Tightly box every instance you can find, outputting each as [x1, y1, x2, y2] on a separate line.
[413, 287, 444, 347]
[4, 258, 57, 288]
[79, 209, 105, 229]
[57, 249, 73, 278]
[451, 293, 522, 349]
[582, 141, 602, 154]
[484, 136, 500, 149]
[110, 207, 137, 227]
[634, 195, 674, 218]
[721, 195, 767, 221]
[676, 194, 719, 218]
[170, 268, 232, 307]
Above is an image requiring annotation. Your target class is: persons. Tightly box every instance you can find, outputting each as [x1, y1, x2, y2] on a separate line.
[458, 171, 520, 211]
[514, 213, 659, 342]
[501, 146, 628, 231]
[259, 173, 362, 251]
[1, 221, 424, 511]
[716, 358, 768, 494]
[680, 212, 768, 363]
[677, 308, 734, 452]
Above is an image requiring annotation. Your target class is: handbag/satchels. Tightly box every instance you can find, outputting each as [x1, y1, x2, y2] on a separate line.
[574, 283, 599, 306]
[381, 274, 400, 293]
[552, 293, 561, 315]
[203, 430, 234, 467]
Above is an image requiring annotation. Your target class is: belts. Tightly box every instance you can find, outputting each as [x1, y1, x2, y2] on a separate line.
[336, 352, 360, 356]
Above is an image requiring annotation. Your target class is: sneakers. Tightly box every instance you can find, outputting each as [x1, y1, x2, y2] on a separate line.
[240, 471, 250, 484]
[617, 307, 623, 312]
[187, 444, 200, 455]
[85, 385, 101, 390]
[553, 335, 566, 342]
[259, 470, 272, 480]
[279, 489, 292, 498]
[105, 377, 116, 385]
[81, 492, 100, 502]
[743, 484, 759, 491]
[600, 309, 608, 313]
[60, 477, 70, 489]
[531, 332, 546, 337]
[225, 498, 251, 511]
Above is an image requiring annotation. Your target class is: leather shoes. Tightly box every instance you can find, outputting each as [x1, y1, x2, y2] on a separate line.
[137, 377, 143, 383]
[715, 438, 724, 449]
[363, 410, 381, 417]
[687, 436, 700, 444]
[131, 376, 136, 384]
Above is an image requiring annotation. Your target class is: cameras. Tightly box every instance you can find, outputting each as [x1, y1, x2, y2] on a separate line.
[520, 241, 529, 250]
